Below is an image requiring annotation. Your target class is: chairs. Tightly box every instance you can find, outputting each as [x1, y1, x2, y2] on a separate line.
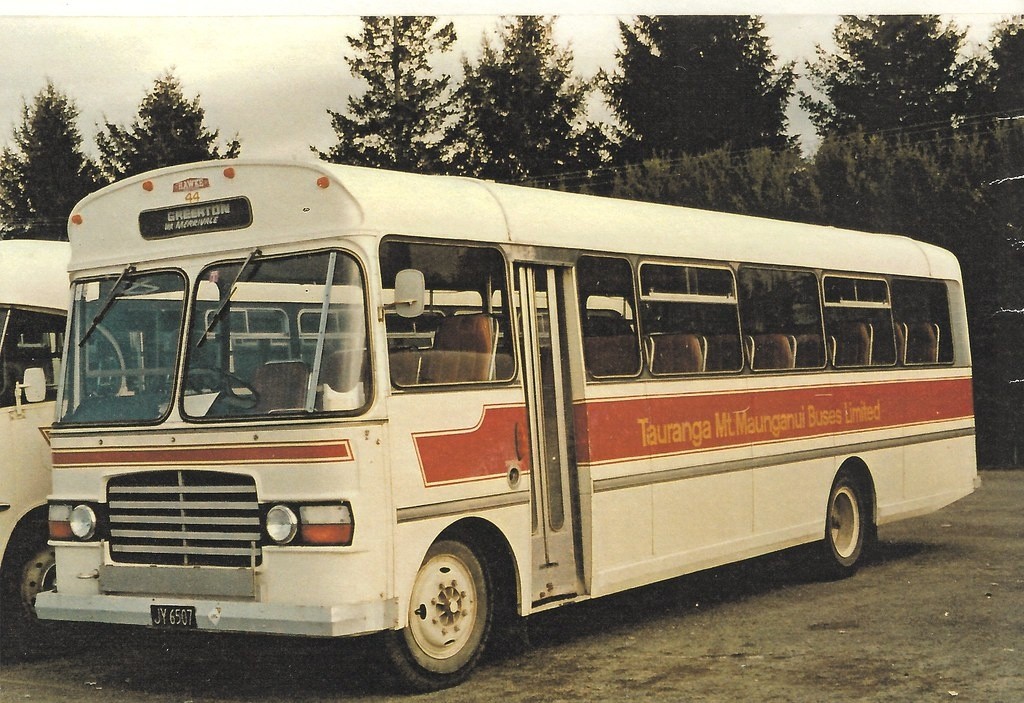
[5, 308, 951, 402]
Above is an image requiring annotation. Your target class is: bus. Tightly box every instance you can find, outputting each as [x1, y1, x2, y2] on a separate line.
[35, 154, 979, 691]
[0, 240, 634, 663]
